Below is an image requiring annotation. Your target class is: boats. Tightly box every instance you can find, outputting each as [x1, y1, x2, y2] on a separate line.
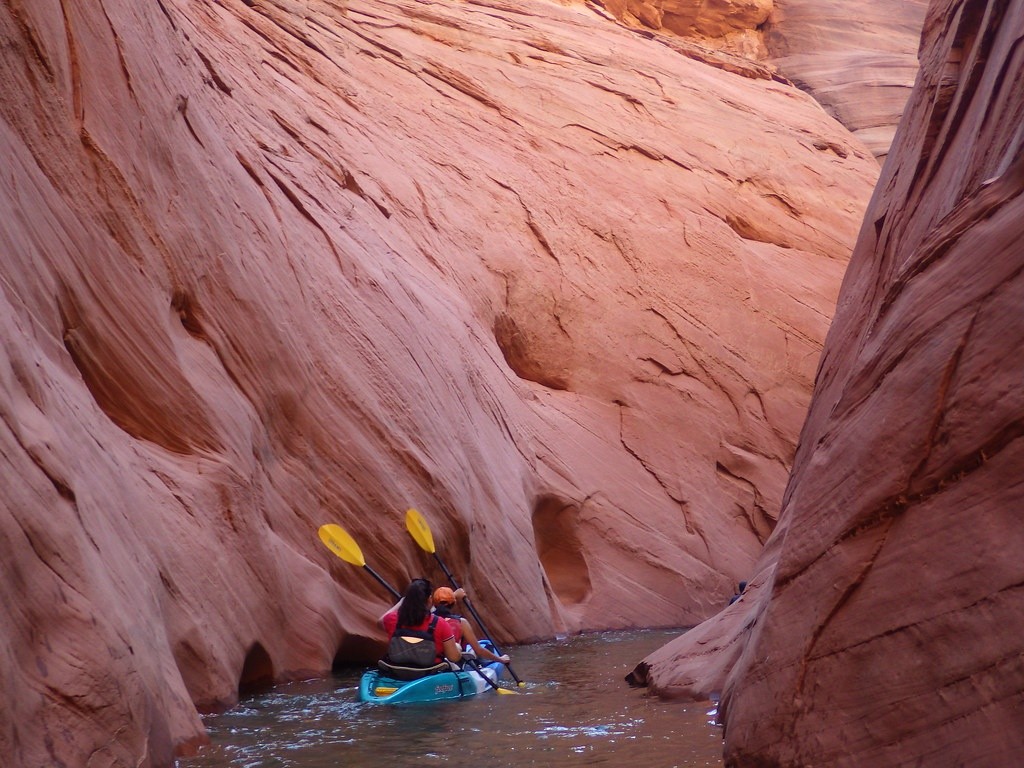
[359, 637, 506, 706]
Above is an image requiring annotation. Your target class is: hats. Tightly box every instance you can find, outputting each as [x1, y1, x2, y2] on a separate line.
[432, 587, 456, 607]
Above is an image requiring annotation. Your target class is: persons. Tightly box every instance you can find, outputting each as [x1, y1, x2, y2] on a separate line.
[729, 581, 747, 604]
[432, 586, 510, 663]
[377, 579, 461, 670]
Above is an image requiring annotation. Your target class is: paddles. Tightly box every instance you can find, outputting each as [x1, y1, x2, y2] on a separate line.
[406, 506, 524, 685]
[317, 522, 515, 693]
